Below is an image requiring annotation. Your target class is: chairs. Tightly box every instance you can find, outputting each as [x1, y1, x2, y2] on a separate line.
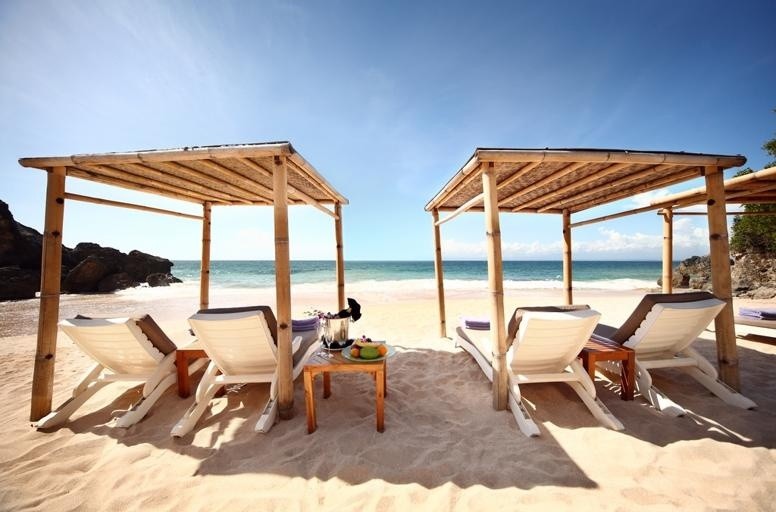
[453, 304, 626, 438]
[32, 313, 210, 430]
[170, 305, 320, 439]
[594, 291, 759, 416]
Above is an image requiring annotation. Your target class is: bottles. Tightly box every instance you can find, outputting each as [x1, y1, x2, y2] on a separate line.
[332, 307, 353, 319]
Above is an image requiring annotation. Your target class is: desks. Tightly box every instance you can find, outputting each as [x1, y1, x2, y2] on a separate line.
[300, 335, 392, 438]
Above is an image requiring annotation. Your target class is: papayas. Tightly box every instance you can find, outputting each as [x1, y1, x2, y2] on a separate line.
[359, 348, 379, 359]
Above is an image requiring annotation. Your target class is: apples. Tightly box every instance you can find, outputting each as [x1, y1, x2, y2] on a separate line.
[351, 345, 360, 350]
[350, 348, 360, 357]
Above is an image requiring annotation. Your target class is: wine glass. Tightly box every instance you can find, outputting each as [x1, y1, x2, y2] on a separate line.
[316, 327, 327, 357]
[321, 326, 335, 359]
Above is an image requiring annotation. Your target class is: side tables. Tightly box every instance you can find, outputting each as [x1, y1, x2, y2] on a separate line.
[175, 338, 226, 399]
[577, 335, 636, 402]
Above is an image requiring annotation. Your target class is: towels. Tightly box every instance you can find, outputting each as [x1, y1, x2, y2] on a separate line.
[737, 302, 776, 320]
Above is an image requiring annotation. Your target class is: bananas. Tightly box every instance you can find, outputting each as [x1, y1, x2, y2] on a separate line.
[356, 340, 380, 347]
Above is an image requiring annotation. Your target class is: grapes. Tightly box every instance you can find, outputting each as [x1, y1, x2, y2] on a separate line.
[361, 335, 372, 343]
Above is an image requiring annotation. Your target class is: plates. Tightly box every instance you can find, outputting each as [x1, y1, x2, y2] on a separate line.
[341, 343, 396, 362]
[320, 338, 356, 352]
